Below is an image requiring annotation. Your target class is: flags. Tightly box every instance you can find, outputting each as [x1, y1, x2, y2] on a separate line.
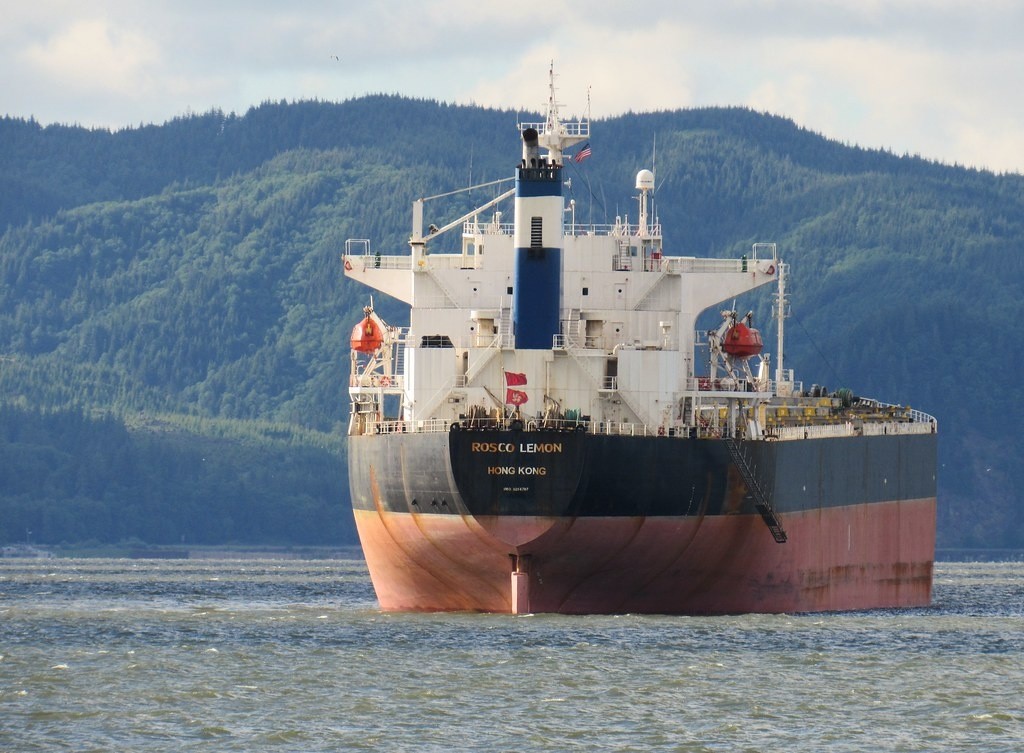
[506, 388, 528, 406]
[574, 142, 592, 163]
[505, 371, 527, 386]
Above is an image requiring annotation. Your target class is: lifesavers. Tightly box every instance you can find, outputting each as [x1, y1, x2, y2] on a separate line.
[344, 261, 352, 271]
[767, 266, 775, 275]
[380, 376, 392, 387]
[658, 427, 665, 434]
[700, 379, 712, 391]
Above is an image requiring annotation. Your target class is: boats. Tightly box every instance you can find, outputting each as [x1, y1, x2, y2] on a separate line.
[340, 54, 938, 615]
